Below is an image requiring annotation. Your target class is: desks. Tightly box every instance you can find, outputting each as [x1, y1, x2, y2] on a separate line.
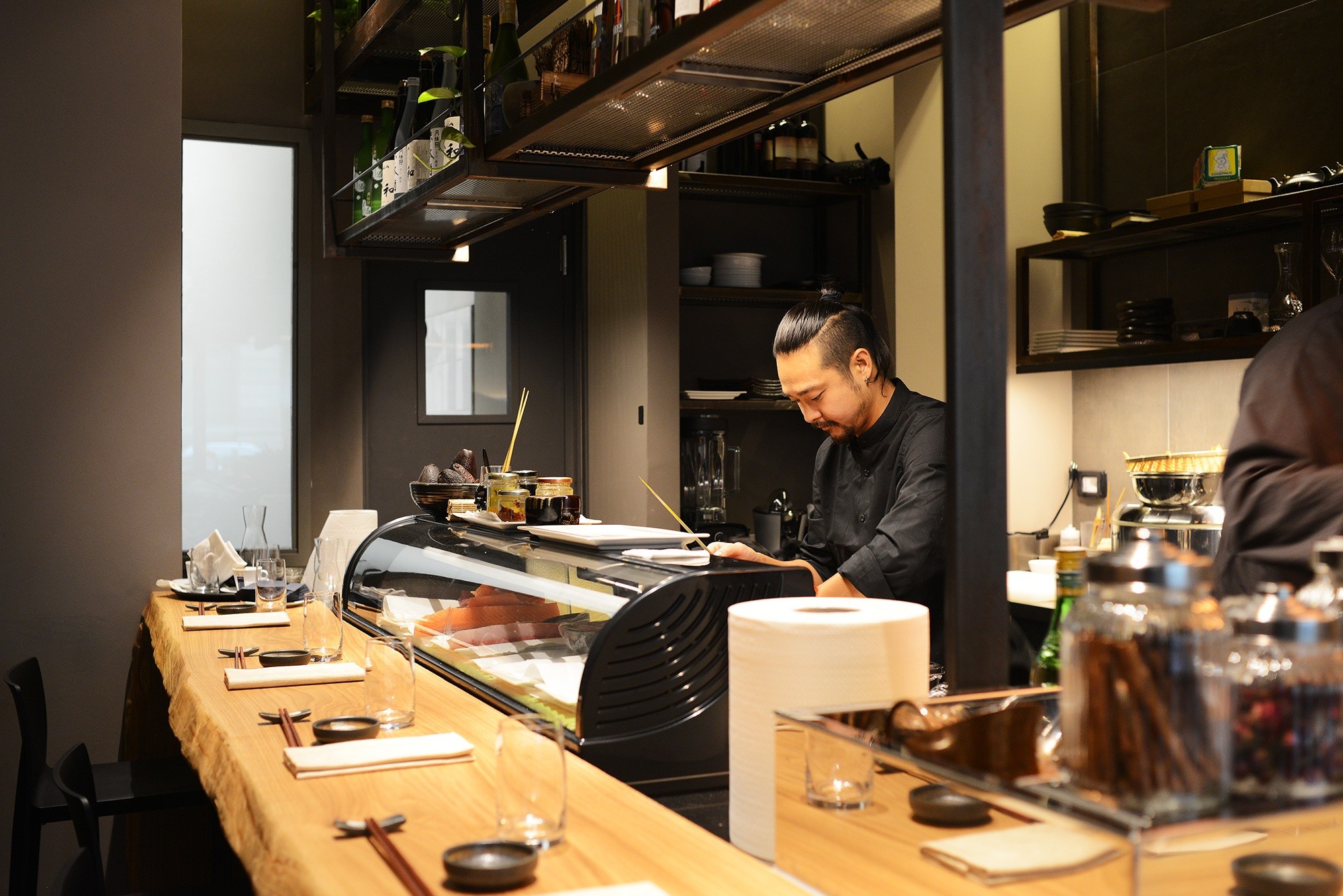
[111, 589, 820, 896]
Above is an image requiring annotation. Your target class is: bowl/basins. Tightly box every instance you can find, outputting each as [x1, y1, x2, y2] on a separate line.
[409, 481, 479, 520]
[679, 267, 712, 286]
[1130, 472, 1222, 506]
[542, 612, 610, 656]
[1042, 201, 1108, 237]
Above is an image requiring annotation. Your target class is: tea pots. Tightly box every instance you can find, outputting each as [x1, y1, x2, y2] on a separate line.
[1268, 161, 1343, 197]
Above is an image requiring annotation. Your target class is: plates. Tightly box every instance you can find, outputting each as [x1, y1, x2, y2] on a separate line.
[216, 603, 536, 891]
[711, 252, 767, 289]
[1029, 297, 1173, 355]
[680, 374, 785, 399]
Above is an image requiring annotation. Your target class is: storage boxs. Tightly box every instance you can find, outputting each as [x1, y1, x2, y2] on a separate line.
[1146, 191, 1194, 219]
[1194, 178, 1272, 213]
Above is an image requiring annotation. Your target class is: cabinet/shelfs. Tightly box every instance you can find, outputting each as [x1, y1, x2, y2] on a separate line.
[587, 163, 875, 530]
[298, 0, 1078, 263]
[1016, 177, 1343, 374]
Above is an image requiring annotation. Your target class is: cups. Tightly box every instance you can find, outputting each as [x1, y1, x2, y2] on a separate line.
[480, 465, 512, 488]
[185, 547, 268, 594]
[751, 504, 808, 556]
[252, 543, 566, 847]
[1080, 521, 1105, 557]
[1028, 559, 1057, 573]
[805, 704, 878, 809]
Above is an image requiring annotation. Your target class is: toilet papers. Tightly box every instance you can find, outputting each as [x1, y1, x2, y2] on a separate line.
[724, 593, 932, 865]
[297, 508, 379, 604]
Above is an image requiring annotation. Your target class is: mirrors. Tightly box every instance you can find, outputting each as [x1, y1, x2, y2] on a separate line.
[417, 279, 519, 423]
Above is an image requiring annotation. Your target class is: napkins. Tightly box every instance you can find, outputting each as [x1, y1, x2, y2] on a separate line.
[182, 611, 290, 631]
[187, 529, 247, 585]
[283, 732, 474, 781]
[224, 662, 365, 690]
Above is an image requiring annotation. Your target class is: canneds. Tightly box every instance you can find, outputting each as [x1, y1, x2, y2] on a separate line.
[485, 470, 574, 522]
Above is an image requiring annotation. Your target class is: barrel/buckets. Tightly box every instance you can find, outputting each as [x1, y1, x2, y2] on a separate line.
[1109, 502, 1224, 560]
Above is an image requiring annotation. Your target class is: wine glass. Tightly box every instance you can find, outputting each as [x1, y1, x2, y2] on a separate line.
[1320, 227, 1343, 297]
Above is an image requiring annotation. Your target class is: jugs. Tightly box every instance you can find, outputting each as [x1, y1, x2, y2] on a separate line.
[679, 413, 740, 524]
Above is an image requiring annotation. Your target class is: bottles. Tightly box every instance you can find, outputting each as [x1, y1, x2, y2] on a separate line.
[1030, 546, 1088, 688]
[1058, 528, 1342, 829]
[352, 0, 818, 223]
[1254, 241, 1306, 333]
[1060, 523, 1080, 546]
[239, 505, 341, 594]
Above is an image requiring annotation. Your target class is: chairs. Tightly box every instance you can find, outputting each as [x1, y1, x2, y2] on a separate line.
[2, 658, 210, 896]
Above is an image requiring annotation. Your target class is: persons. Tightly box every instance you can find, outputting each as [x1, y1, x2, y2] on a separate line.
[1210, 295, 1343, 601]
[702, 278, 1040, 690]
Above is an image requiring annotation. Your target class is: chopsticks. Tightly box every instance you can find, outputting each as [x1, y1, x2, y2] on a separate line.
[500, 387, 529, 473]
[198, 602, 205, 615]
[278, 707, 303, 748]
[234, 646, 246, 669]
[365, 817, 434, 896]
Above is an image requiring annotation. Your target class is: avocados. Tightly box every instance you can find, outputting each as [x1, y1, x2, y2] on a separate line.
[417, 448, 480, 484]
